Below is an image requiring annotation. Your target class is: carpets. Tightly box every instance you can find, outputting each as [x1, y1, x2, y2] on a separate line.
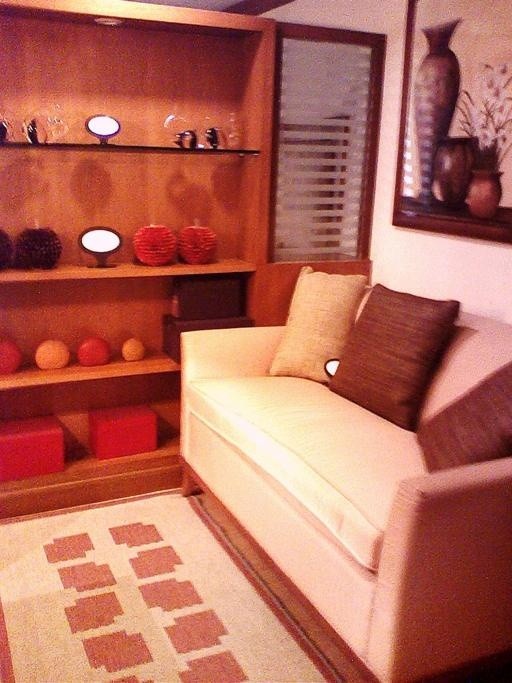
[0, 487, 343, 683]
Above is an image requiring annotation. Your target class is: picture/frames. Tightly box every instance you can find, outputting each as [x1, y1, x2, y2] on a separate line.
[390, 0, 511, 251]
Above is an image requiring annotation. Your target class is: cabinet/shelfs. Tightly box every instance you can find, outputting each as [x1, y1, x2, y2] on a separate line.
[2, 0, 277, 516]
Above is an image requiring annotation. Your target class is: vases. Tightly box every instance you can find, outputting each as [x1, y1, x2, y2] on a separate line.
[463, 168, 505, 220]
[432, 135, 482, 207]
[413, 14, 463, 202]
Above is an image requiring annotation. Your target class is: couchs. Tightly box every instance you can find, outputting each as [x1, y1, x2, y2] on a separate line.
[175, 267, 512, 682]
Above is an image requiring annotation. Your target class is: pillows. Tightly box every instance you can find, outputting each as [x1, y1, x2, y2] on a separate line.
[326, 282, 462, 434]
[270, 264, 370, 388]
[414, 358, 511, 477]
